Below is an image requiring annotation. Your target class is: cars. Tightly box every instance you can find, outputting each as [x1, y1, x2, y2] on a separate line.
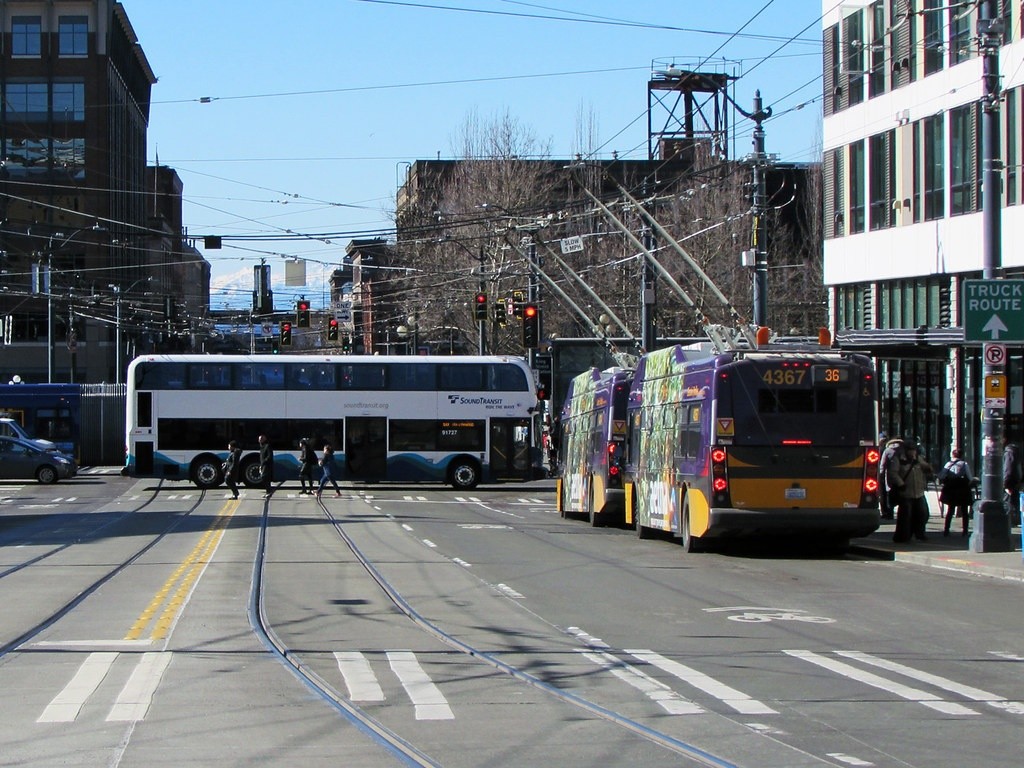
[0, 435, 78, 485]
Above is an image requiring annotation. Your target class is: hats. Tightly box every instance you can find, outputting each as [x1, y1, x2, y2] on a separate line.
[301, 438, 309, 446]
[879, 433, 887, 441]
[229, 440, 236, 448]
[904, 440, 916, 448]
[953, 449, 961, 458]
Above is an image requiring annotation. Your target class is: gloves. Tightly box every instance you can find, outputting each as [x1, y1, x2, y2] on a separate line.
[900, 484, 907, 492]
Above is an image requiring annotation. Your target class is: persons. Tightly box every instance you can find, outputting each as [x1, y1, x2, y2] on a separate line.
[314, 445, 342, 497]
[941, 449, 979, 537]
[876, 432, 932, 544]
[221, 440, 242, 500]
[298, 438, 316, 495]
[257, 434, 274, 500]
[1001, 433, 1023, 526]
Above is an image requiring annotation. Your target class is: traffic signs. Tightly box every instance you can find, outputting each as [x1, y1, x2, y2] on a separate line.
[334, 309, 352, 322]
[962, 279, 1024, 345]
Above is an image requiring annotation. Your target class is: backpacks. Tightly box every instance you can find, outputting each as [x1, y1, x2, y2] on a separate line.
[309, 449, 318, 466]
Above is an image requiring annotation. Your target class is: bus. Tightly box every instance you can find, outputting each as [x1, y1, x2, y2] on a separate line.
[120, 354, 547, 489]
[554, 368, 636, 529]
[625, 327, 884, 555]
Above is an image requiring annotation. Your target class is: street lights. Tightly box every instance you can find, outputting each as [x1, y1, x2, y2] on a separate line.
[114, 274, 161, 383]
[47, 225, 109, 383]
[433, 237, 485, 356]
[561, 163, 654, 355]
[474, 202, 536, 369]
[665, 66, 770, 331]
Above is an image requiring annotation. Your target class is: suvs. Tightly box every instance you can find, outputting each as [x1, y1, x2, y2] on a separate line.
[0, 418, 56, 451]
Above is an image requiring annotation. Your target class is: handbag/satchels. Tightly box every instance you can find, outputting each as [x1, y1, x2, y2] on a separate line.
[938, 469, 956, 484]
[888, 484, 900, 507]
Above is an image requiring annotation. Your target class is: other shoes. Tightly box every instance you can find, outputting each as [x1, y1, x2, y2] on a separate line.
[881, 514, 894, 520]
[335, 492, 341, 497]
[916, 534, 927, 541]
[306, 491, 314, 494]
[263, 494, 272, 498]
[229, 493, 239, 500]
[314, 493, 322, 496]
[299, 490, 307, 494]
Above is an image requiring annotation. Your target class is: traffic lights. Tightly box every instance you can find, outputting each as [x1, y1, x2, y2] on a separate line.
[512, 292, 523, 319]
[522, 301, 538, 348]
[329, 317, 339, 340]
[342, 337, 350, 351]
[296, 300, 312, 328]
[496, 299, 506, 328]
[476, 292, 487, 321]
[280, 320, 292, 346]
